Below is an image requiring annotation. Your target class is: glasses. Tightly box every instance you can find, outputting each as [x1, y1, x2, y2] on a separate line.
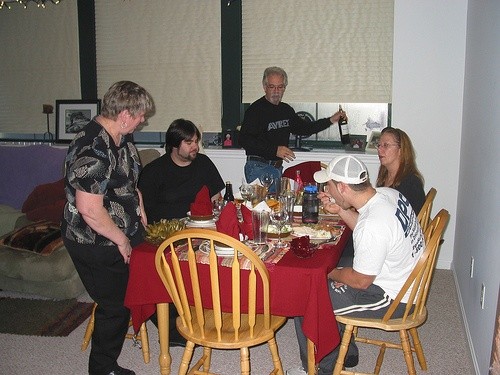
[264, 83, 285, 91]
[375, 143, 399, 149]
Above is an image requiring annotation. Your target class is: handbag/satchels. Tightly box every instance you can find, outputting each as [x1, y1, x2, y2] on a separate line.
[215, 201, 259, 243]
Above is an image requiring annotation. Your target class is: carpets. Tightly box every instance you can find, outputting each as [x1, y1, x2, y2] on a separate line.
[0, 297, 95, 336]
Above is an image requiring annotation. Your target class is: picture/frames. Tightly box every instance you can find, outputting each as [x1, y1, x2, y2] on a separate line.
[365, 132, 381, 153]
[56, 100, 101, 144]
[346, 135, 366, 151]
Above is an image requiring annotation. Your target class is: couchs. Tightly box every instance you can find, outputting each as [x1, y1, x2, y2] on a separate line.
[0, 144, 161, 300]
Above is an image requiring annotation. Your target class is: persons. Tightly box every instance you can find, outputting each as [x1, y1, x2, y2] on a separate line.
[132, 117, 229, 247]
[53, 79, 201, 375]
[364, 127, 428, 226]
[286, 154, 427, 375]
[239, 67, 349, 203]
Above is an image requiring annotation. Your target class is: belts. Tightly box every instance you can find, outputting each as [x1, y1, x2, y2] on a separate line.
[246, 157, 283, 168]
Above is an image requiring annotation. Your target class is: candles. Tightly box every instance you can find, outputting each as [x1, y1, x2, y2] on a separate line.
[43, 104, 53, 112]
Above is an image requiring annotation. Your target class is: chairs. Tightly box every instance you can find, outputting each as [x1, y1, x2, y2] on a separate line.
[416, 187, 437, 233]
[335, 209, 448, 375]
[155, 228, 287, 375]
[283, 161, 328, 185]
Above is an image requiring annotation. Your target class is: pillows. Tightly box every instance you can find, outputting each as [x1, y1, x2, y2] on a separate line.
[0, 178, 67, 256]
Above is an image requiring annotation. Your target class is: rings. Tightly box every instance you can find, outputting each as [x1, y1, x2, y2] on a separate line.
[126, 254, 130, 257]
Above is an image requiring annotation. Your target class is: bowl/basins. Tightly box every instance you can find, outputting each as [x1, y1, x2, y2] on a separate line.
[186, 210, 215, 220]
[289, 241, 319, 258]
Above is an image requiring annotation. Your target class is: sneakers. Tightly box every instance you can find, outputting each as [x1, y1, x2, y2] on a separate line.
[286, 365, 308, 375]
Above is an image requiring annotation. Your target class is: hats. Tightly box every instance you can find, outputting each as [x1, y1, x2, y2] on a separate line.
[314, 155, 369, 184]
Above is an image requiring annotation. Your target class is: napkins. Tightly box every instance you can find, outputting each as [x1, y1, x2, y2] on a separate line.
[238, 204, 260, 240]
[216, 202, 240, 241]
[190, 186, 213, 215]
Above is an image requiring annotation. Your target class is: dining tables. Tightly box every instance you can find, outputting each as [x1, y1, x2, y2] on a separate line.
[124, 200, 353, 375]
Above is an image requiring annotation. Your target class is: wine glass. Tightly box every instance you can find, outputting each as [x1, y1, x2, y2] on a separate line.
[261, 173, 274, 192]
[270, 208, 289, 249]
[240, 185, 253, 209]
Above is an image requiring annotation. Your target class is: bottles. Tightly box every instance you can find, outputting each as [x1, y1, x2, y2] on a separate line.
[279, 170, 303, 223]
[338, 104, 350, 144]
[302, 186, 319, 224]
[223, 183, 234, 207]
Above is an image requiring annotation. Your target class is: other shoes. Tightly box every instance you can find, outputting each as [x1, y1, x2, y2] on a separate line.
[158, 329, 198, 348]
[109, 366, 135, 375]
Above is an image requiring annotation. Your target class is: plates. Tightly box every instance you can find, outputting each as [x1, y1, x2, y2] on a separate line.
[290, 231, 330, 244]
[199, 241, 259, 257]
[179, 217, 217, 227]
[259, 224, 292, 234]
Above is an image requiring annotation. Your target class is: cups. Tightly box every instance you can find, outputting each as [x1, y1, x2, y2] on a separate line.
[252, 210, 269, 245]
